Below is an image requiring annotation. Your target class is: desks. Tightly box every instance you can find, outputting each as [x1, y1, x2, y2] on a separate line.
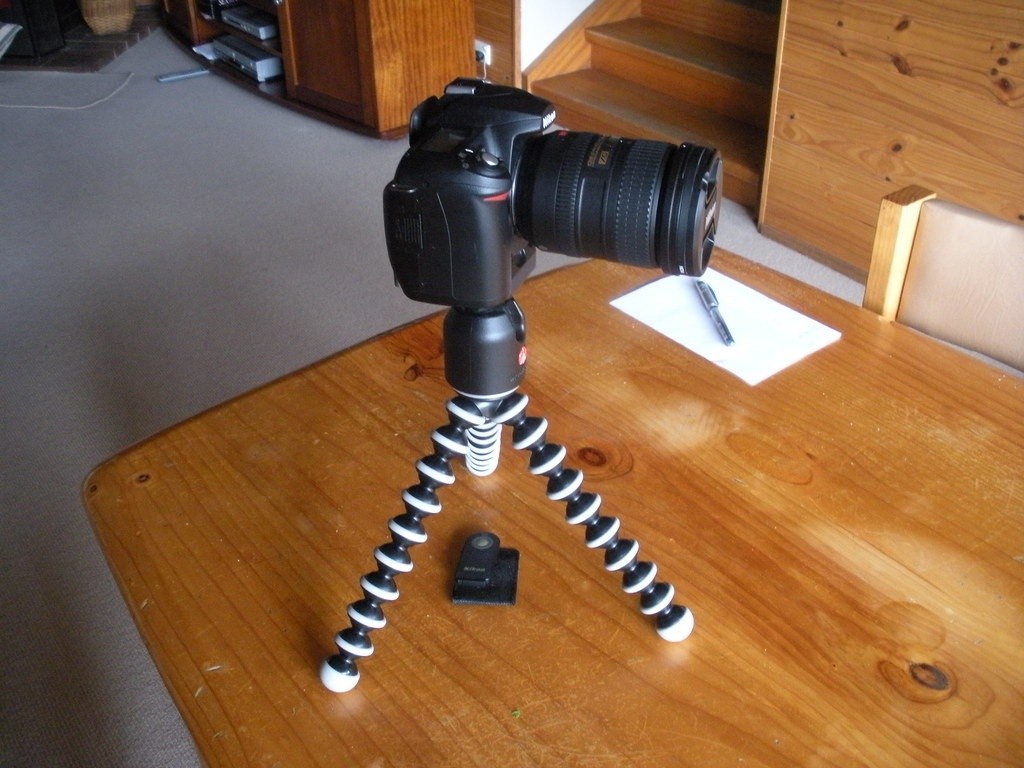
[81, 241, 1024, 768]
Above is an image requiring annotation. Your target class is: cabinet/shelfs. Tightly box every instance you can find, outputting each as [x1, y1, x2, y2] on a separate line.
[156, 0, 476, 142]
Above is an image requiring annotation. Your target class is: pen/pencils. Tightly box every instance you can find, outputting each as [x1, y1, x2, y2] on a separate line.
[694, 279, 733, 347]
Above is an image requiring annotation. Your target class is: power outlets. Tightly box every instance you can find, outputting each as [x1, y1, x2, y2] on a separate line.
[475, 40, 491, 65]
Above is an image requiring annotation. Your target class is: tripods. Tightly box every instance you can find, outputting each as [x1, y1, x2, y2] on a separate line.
[318, 298, 694, 694]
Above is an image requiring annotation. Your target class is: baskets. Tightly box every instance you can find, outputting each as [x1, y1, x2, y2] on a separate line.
[81, 0, 136, 36]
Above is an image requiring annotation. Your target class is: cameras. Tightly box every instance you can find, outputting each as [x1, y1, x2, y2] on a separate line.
[383, 75, 725, 308]
